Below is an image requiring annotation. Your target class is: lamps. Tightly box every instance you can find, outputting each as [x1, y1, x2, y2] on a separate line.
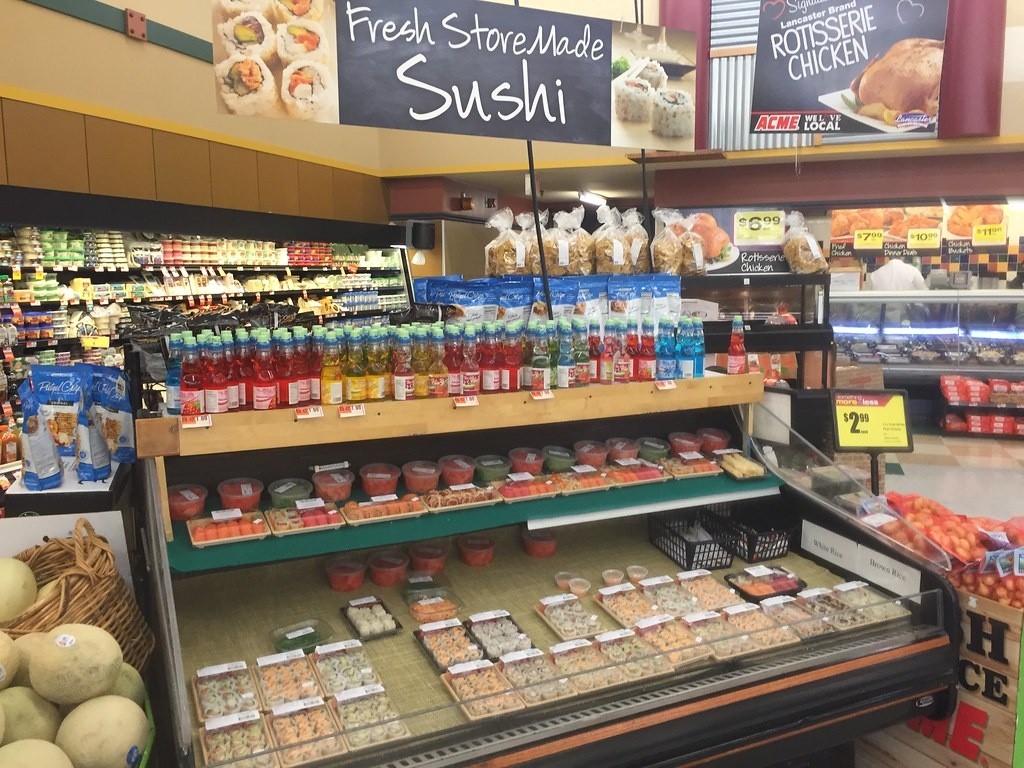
[578, 190, 608, 206]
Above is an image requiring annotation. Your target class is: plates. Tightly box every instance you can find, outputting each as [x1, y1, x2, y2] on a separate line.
[705, 243, 740, 270]
[631, 49, 696, 78]
[818, 88, 937, 133]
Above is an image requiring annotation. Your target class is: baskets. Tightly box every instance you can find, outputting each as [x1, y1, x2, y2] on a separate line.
[700, 501, 789, 564]
[645, 504, 743, 571]
[0, 517, 156, 678]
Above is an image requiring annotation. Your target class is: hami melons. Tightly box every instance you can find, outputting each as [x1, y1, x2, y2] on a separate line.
[0, 557, 151, 768]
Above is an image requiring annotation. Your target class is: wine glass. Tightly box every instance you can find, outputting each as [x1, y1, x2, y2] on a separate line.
[624, 23, 679, 54]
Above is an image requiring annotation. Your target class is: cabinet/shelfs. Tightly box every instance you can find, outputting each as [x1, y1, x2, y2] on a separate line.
[134, 367, 785, 573]
[0, 266, 409, 474]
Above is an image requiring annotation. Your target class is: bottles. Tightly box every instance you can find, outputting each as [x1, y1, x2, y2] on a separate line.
[166, 315, 747, 416]
[0, 418, 24, 465]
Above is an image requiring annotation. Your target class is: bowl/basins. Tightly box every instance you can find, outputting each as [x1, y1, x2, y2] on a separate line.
[522, 525, 559, 558]
[944, 351, 971, 364]
[555, 571, 576, 591]
[327, 555, 367, 591]
[217, 467, 355, 513]
[626, 565, 649, 583]
[911, 351, 941, 364]
[409, 542, 450, 574]
[975, 352, 1005, 365]
[167, 484, 208, 521]
[360, 427, 732, 496]
[458, 531, 496, 566]
[568, 577, 591, 597]
[367, 551, 410, 587]
[602, 569, 624, 587]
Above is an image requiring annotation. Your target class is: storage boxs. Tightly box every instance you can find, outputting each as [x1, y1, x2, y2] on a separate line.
[822, 450, 886, 495]
[883, 590, 1024, 768]
[190, 566, 913, 768]
[836, 357, 885, 390]
